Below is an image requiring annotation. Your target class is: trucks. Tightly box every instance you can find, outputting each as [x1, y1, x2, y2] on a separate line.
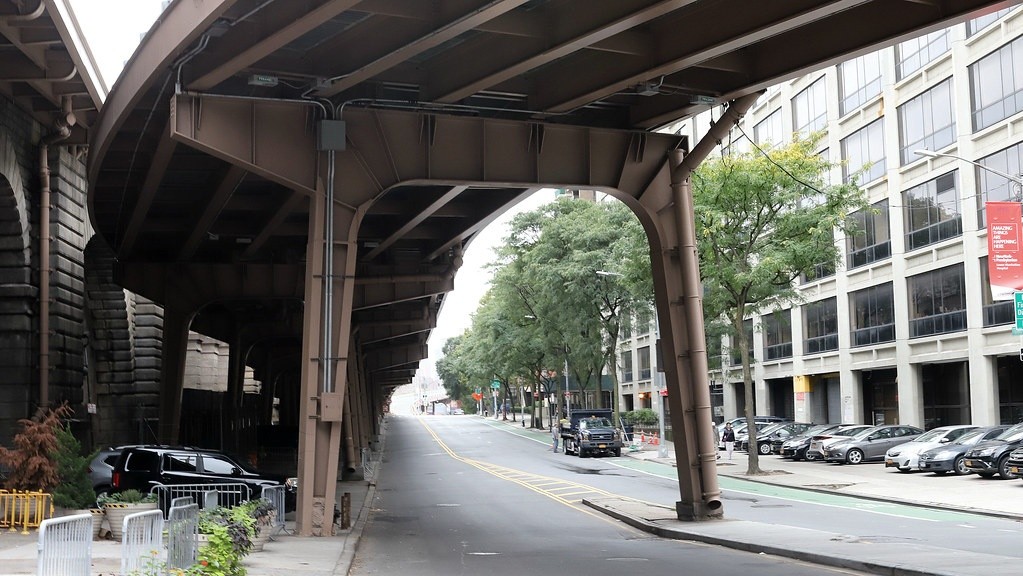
[560, 408, 622, 458]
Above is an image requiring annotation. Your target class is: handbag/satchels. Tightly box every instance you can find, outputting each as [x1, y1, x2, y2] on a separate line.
[724, 438, 726, 442]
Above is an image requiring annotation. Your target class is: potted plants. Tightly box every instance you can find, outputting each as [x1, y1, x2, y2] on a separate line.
[189, 497, 279, 557]
[46, 421, 105, 541]
[98, 487, 158, 542]
[0, 399, 76, 522]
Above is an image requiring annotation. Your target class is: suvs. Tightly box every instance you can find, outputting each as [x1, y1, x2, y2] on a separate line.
[111, 447, 299, 519]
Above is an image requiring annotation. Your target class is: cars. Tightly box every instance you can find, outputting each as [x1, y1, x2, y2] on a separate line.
[809, 425, 876, 463]
[715, 415, 817, 455]
[918, 425, 1015, 475]
[824, 425, 927, 465]
[963, 422, 1023, 480]
[1007, 445, 1023, 480]
[86, 445, 194, 509]
[884, 425, 982, 472]
[780, 424, 855, 461]
[454, 409, 464, 415]
[499, 404, 510, 413]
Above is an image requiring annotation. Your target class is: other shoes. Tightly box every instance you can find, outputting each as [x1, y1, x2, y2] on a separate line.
[717, 456, 720, 459]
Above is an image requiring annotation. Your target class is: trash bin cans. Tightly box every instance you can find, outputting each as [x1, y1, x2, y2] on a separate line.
[535, 416, 540, 428]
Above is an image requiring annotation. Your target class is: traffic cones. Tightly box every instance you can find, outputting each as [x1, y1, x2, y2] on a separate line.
[653, 432, 660, 445]
[648, 432, 653, 444]
[640, 430, 646, 442]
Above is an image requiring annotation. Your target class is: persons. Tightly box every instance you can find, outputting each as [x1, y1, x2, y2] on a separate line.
[722, 422, 735, 459]
[712, 422, 721, 459]
[552, 424, 559, 453]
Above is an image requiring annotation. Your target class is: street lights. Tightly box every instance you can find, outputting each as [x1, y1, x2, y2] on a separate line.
[595, 270, 669, 458]
[524, 315, 570, 420]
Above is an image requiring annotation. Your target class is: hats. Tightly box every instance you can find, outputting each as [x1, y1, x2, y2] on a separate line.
[712, 422, 715, 426]
